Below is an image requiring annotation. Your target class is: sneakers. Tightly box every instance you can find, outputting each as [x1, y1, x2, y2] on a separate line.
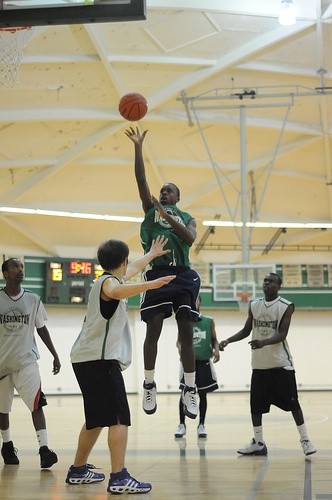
[197, 437, 207, 448]
[1, 440, 19, 464]
[175, 437, 186, 448]
[181, 385, 200, 419]
[38, 446, 57, 468]
[237, 438, 268, 455]
[66, 464, 105, 485]
[107, 468, 152, 494]
[301, 440, 316, 456]
[142, 380, 157, 414]
[174, 423, 186, 437]
[197, 425, 208, 437]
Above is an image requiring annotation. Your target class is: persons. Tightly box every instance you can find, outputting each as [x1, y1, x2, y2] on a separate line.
[175, 296, 219, 438]
[220, 272, 318, 456]
[0, 258, 62, 467]
[126, 125, 201, 418]
[64, 235, 176, 495]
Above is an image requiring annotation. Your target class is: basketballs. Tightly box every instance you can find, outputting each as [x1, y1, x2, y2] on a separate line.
[118, 92, 147, 122]
